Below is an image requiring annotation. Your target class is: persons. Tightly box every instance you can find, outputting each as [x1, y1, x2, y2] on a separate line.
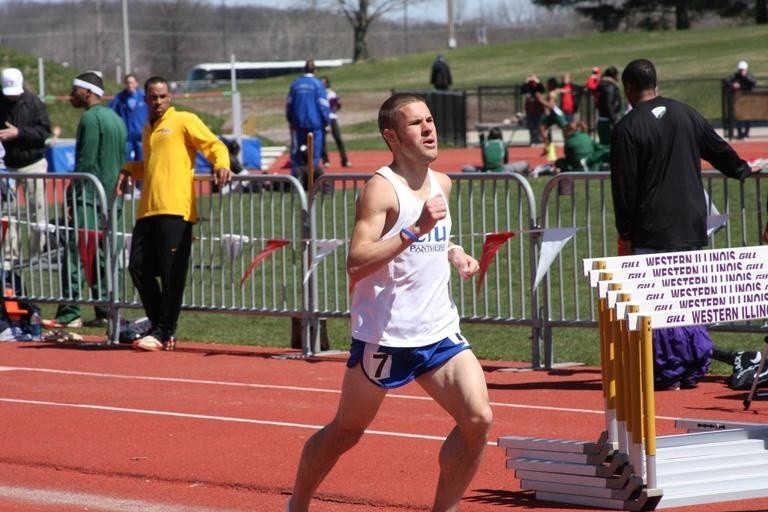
[611, 59, 763, 390]
[726, 61, 756, 139]
[0, 67, 50, 251]
[286, 60, 330, 178]
[41, 73, 128, 329]
[520, 66, 622, 171]
[484, 127, 509, 173]
[115, 77, 232, 351]
[288, 93, 493, 512]
[108, 75, 151, 200]
[319, 76, 351, 168]
[431, 54, 452, 90]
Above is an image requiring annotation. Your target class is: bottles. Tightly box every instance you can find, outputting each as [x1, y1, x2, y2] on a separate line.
[30, 308, 41, 342]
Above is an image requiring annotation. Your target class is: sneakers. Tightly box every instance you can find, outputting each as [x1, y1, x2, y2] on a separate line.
[84, 318, 108, 327]
[133, 334, 164, 351]
[164, 336, 176, 350]
[41, 317, 82, 328]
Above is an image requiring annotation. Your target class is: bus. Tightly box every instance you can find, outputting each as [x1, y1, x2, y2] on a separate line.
[189, 58, 352, 80]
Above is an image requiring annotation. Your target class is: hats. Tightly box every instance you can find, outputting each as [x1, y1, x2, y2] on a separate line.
[737, 61, 747, 69]
[73, 71, 105, 97]
[0, 68, 24, 97]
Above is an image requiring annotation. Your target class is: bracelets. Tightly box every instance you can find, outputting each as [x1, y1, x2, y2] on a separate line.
[400, 228, 425, 243]
